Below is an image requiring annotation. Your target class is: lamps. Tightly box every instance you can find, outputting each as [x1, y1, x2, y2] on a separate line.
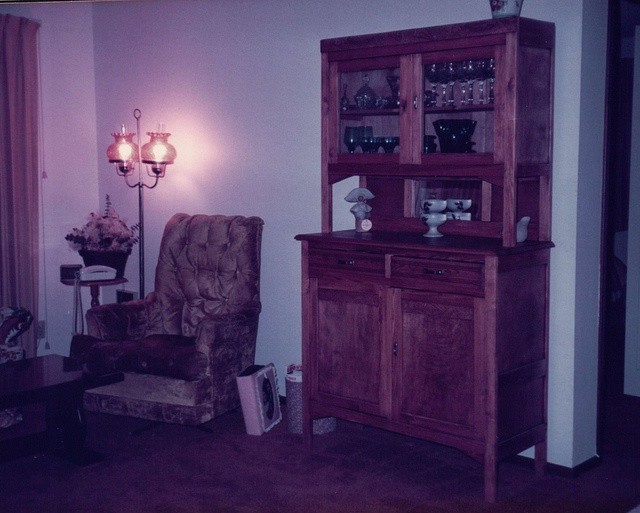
[107, 108, 175, 298]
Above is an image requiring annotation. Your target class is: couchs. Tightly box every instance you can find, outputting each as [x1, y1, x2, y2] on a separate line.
[83, 213, 264, 436]
[0, 305, 34, 429]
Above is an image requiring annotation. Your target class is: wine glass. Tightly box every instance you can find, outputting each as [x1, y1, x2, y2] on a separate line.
[424, 58, 494, 107]
[343, 125, 399, 154]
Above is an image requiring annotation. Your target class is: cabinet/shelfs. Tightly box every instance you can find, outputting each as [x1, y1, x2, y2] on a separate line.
[294, 234, 555, 503]
[320, 15, 555, 247]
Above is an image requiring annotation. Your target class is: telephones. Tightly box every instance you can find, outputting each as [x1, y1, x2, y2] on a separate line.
[75, 265, 117, 281]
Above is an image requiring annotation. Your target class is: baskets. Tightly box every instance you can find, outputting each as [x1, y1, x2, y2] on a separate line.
[77, 217, 132, 278]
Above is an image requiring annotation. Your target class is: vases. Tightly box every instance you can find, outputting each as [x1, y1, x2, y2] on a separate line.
[78, 249, 132, 277]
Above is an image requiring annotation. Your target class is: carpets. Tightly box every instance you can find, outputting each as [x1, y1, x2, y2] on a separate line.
[0, 428, 502, 513]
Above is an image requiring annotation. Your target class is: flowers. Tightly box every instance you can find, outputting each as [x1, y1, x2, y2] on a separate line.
[64, 194, 139, 254]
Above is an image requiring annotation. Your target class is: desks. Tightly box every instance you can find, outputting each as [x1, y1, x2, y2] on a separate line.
[62, 276, 128, 306]
